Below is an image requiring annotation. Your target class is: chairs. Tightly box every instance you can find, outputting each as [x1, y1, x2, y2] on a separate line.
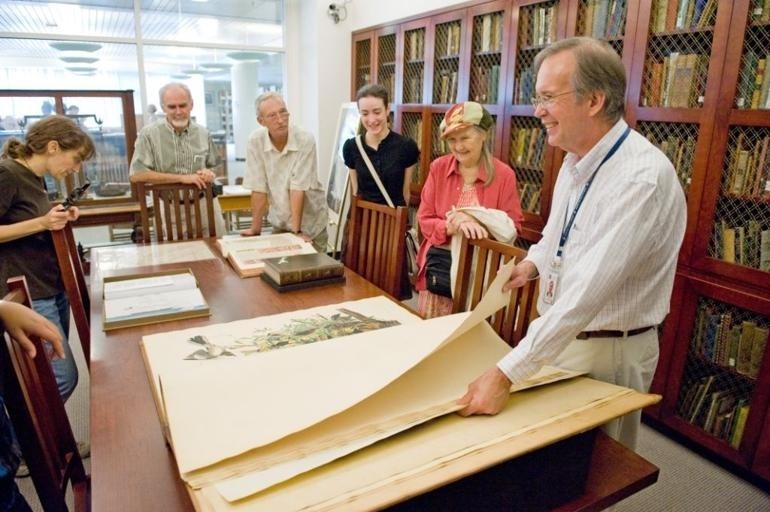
[51, 210, 90, 373]
[0, 275, 91, 512]
[137, 181, 216, 242]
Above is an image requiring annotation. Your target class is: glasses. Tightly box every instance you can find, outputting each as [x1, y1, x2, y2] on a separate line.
[160, 102, 188, 109]
[62, 182, 90, 210]
[530, 90, 573, 109]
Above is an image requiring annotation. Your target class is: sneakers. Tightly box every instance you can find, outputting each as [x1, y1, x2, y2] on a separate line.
[75, 441, 90, 459]
[15, 464, 30, 477]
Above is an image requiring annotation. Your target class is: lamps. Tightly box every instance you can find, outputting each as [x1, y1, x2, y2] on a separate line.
[49, 29, 268, 79]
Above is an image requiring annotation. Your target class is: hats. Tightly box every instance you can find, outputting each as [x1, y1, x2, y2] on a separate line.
[439, 101, 493, 140]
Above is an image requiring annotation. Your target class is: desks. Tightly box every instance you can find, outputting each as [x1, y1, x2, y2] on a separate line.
[218, 185, 269, 233]
[49, 191, 156, 243]
[90, 230, 659, 512]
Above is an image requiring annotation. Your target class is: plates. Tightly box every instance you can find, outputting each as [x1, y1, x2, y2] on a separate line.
[92, 190, 130, 201]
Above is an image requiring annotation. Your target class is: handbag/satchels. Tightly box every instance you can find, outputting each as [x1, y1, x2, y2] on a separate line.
[425, 246, 452, 298]
[404, 227, 420, 286]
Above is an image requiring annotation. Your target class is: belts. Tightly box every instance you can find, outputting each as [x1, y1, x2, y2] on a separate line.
[576, 327, 651, 340]
[169, 192, 204, 204]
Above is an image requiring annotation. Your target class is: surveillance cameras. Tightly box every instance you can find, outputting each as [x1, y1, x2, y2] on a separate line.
[328, 2, 338, 13]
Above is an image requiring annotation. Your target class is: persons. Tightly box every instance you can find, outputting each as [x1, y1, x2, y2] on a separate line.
[0, 114, 95, 477]
[341, 82, 419, 301]
[238, 93, 329, 257]
[459, 34, 688, 452]
[0, 300, 69, 511]
[418, 99, 526, 325]
[126, 82, 233, 237]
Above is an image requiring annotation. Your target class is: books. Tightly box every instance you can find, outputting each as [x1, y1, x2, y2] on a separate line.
[350, 1, 770, 272]
[102, 267, 213, 332]
[137, 293, 664, 512]
[691, 298, 770, 382]
[216, 230, 346, 293]
[679, 370, 751, 450]
